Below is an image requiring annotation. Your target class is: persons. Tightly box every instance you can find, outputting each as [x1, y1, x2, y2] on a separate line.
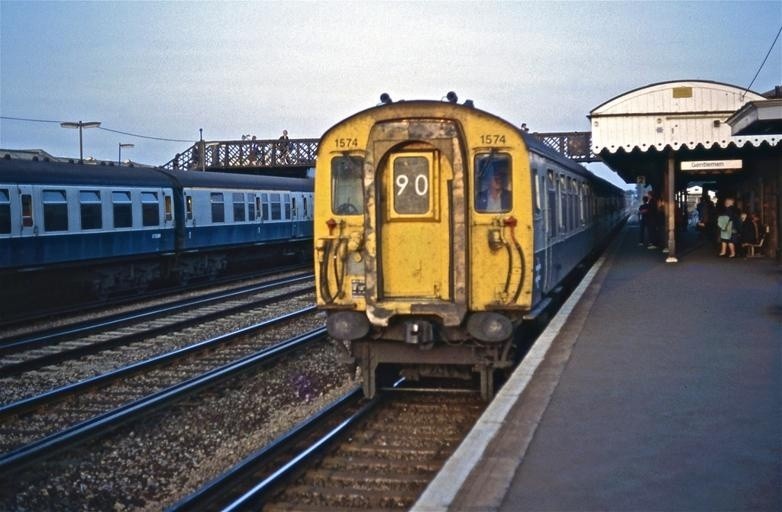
[173, 153, 182, 170]
[478, 169, 511, 211]
[191, 141, 201, 166]
[248, 136, 263, 165]
[521, 123, 529, 133]
[636, 188, 768, 258]
[277, 129, 294, 166]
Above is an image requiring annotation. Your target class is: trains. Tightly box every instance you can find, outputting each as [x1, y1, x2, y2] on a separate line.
[1, 154, 315, 303]
[314, 92, 634, 403]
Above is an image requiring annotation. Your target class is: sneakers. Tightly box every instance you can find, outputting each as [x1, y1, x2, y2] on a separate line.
[638, 242, 657, 249]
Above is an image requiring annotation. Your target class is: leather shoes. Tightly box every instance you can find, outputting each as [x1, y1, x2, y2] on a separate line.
[718, 252, 736, 258]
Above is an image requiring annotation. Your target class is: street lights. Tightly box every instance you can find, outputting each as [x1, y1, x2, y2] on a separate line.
[61, 121, 101, 164]
[117, 142, 135, 165]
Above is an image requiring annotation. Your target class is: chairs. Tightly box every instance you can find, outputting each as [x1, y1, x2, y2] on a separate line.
[741, 223, 769, 258]
